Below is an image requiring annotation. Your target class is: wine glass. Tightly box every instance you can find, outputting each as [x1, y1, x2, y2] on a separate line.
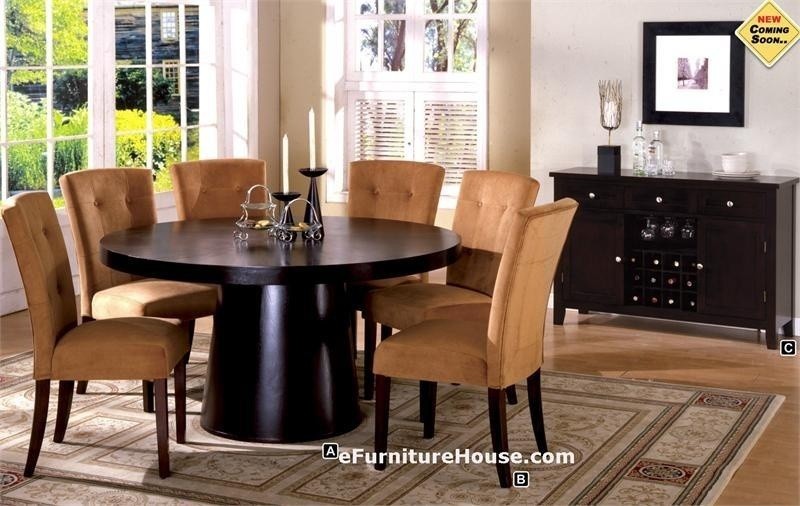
[640, 214, 695, 240]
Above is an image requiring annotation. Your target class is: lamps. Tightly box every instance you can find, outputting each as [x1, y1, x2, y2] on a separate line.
[597, 77, 623, 174]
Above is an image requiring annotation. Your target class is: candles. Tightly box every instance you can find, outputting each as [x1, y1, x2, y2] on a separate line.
[308, 111, 316, 169]
[282, 136, 289, 194]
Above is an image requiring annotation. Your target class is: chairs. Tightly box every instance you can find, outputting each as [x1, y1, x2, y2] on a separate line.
[1, 157, 580, 497]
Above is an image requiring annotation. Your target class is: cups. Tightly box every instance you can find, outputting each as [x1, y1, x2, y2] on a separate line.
[661, 158, 675, 177]
[720, 152, 747, 172]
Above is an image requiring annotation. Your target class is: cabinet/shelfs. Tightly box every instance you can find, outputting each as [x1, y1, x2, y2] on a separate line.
[542, 165, 800, 352]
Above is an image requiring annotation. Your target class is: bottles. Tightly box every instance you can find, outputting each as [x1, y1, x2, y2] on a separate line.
[631, 120, 647, 177]
[647, 128, 666, 176]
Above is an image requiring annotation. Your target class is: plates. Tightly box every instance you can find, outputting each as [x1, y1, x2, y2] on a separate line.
[712, 169, 763, 181]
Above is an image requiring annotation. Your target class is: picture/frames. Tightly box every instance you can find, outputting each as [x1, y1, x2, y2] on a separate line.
[640, 20, 747, 128]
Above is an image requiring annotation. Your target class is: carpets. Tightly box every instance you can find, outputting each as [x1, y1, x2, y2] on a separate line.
[1, 334, 786, 505]
